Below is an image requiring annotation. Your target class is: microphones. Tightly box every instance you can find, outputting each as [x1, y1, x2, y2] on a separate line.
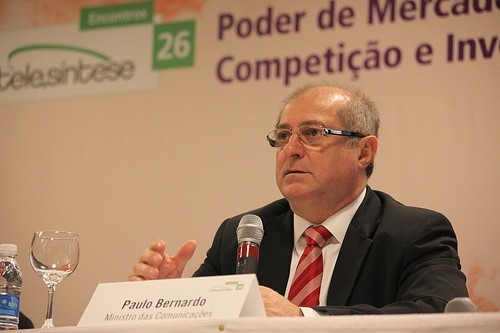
[235, 213, 265, 275]
[444, 297, 479, 314]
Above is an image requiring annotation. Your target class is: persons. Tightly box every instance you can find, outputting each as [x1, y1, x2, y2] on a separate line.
[130, 81, 469, 317]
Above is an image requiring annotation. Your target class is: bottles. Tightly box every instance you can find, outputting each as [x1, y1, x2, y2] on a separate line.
[0, 243, 24, 331]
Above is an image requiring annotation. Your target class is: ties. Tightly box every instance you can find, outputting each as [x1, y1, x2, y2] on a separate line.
[288, 225, 334, 308]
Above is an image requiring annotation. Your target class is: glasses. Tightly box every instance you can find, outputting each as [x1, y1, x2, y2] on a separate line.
[266, 126, 365, 150]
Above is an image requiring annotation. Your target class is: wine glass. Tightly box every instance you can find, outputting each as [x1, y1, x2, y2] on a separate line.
[29, 230, 79, 327]
